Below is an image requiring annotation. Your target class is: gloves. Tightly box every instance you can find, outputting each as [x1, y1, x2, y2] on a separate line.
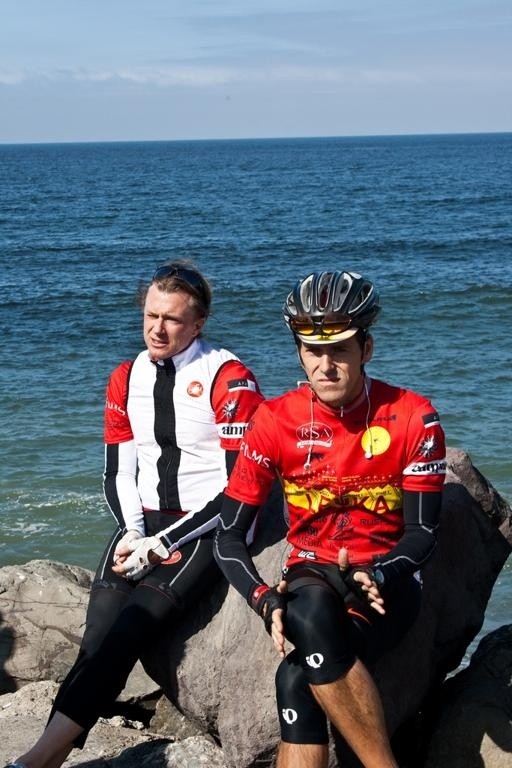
[112, 529, 173, 581]
[335, 563, 389, 615]
[248, 580, 288, 637]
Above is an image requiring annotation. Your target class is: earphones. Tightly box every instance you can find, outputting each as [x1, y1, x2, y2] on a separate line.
[364, 444, 372, 459]
[303, 455, 311, 470]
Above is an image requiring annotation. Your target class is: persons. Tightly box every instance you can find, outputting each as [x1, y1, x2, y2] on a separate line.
[212, 269, 446, 768]
[0, 259, 266, 768]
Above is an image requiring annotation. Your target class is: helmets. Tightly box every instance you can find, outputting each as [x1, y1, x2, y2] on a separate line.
[282, 270, 379, 336]
